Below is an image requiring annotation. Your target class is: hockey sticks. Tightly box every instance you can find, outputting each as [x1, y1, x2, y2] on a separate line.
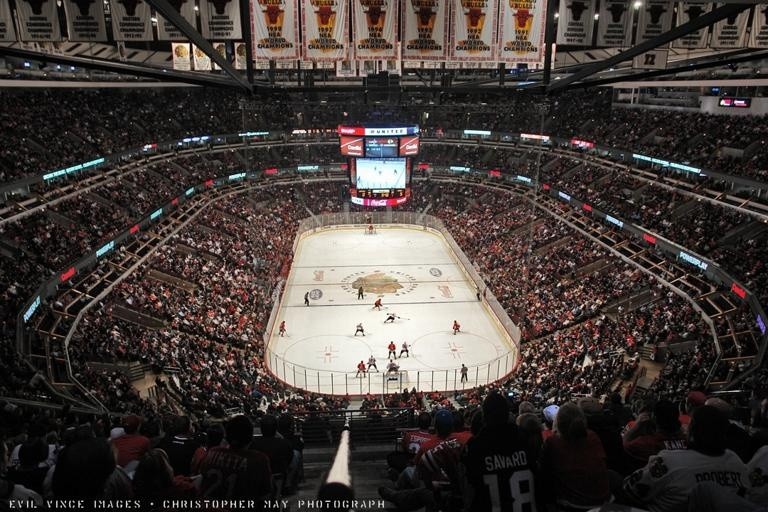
[382, 306, 389, 309]
[285, 331, 291, 338]
[399, 317, 411, 321]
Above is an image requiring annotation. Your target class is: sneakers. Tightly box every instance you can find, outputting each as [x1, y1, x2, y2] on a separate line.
[375, 484, 401, 502]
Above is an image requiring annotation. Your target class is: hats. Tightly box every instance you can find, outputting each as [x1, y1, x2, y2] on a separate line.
[107, 414, 143, 443]
[580, 398, 601, 416]
[689, 388, 730, 413]
[435, 410, 453, 430]
[517, 413, 534, 426]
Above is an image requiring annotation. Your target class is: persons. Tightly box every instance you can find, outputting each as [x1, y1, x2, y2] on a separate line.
[0, 85, 768, 505]
[1, 1, 767, 78]
